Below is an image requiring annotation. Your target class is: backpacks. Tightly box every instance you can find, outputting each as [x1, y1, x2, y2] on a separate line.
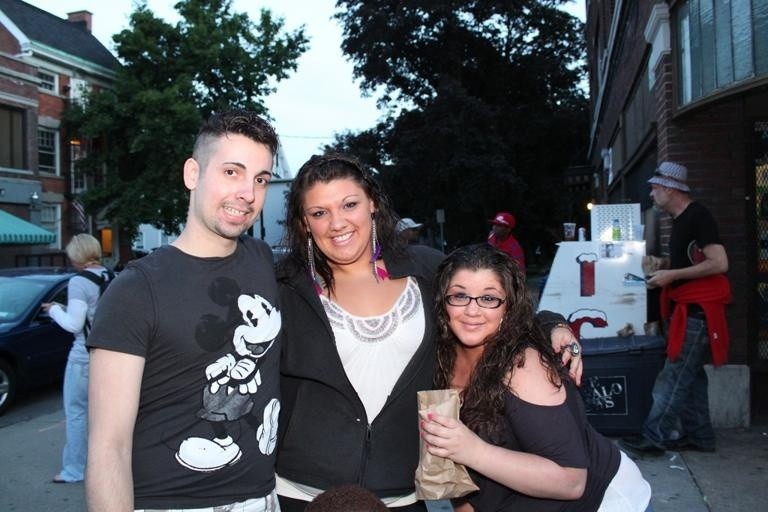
[74, 266, 115, 353]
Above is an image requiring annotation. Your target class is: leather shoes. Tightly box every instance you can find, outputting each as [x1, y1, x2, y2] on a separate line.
[619, 437, 664, 455]
[664, 436, 700, 450]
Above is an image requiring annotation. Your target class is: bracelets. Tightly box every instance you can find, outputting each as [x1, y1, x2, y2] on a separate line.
[552, 322, 575, 334]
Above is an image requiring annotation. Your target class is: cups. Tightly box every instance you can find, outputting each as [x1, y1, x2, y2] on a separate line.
[563, 222, 576, 241]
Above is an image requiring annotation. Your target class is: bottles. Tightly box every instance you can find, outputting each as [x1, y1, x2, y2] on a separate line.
[578, 227, 587, 241]
[612, 218, 621, 240]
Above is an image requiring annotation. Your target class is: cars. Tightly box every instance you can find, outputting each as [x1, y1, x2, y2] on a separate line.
[0, 266, 115, 414]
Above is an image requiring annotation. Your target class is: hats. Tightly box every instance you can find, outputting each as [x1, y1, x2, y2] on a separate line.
[488, 213, 515, 228]
[396, 218, 423, 232]
[648, 161, 691, 192]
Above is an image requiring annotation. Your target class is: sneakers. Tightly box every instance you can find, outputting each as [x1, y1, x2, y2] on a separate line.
[54, 474, 64, 483]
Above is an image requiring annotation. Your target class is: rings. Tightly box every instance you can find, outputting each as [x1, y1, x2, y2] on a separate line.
[562, 341, 580, 357]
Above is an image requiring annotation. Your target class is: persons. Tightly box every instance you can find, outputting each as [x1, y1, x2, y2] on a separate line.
[40, 234, 134, 483]
[423, 240, 637, 510]
[481, 212, 527, 279]
[281, 147, 586, 510]
[79, 115, 284, 510]
[390, 214, 446, 285]
[619, 159, 734, 457]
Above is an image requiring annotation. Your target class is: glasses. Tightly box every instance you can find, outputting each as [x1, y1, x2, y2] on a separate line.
[445, 293, 505, 309]
